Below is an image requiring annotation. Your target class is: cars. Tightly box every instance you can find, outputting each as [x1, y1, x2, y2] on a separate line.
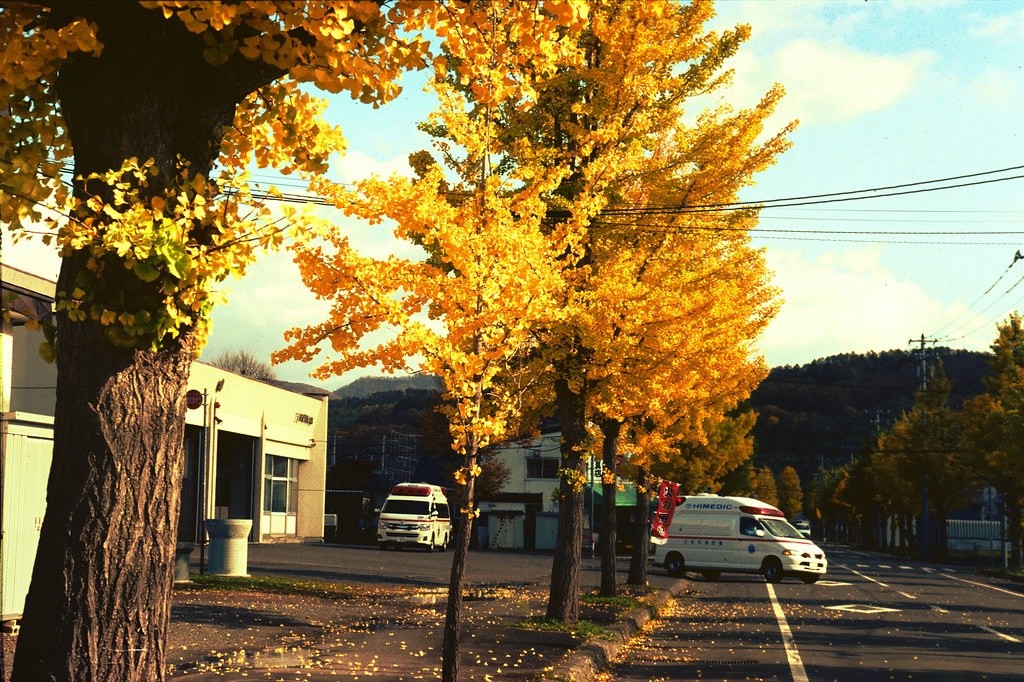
[797, 522, 812, 538]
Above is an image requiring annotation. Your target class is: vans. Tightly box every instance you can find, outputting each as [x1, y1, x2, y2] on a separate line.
[375, 481, 453, 552]
[653, 492, 827, 584]
